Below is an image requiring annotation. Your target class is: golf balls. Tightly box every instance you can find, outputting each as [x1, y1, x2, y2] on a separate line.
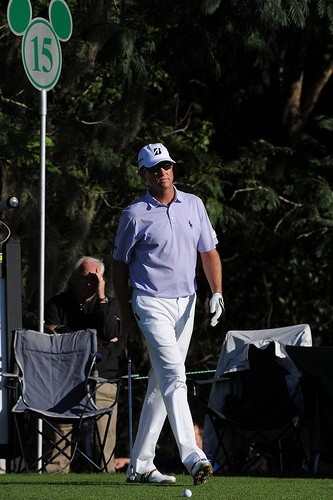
[182, 488, 193, 498]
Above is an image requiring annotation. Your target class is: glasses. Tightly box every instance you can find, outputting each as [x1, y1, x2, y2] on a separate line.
[142, 163, 172, 173]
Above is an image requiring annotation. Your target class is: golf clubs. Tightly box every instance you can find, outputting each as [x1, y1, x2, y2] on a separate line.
[121, 337, 140, 484]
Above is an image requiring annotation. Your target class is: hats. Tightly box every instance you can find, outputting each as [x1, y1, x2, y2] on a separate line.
[137, 142, 177, 171]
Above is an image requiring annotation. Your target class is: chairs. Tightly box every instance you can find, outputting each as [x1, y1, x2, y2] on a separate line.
[11, 328, 115, 475]
[186, 341, 333, 477]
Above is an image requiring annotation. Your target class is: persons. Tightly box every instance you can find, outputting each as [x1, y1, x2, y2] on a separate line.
[45, 256, 123, 474]
[111, 143, 226, 486]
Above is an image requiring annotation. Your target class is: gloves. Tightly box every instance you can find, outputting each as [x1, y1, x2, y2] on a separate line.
[208, 292, 225, 327]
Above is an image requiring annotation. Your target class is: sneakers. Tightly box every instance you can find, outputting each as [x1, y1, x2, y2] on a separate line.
[125, 468, 177, 484]
[190, 457, 213, 486]
